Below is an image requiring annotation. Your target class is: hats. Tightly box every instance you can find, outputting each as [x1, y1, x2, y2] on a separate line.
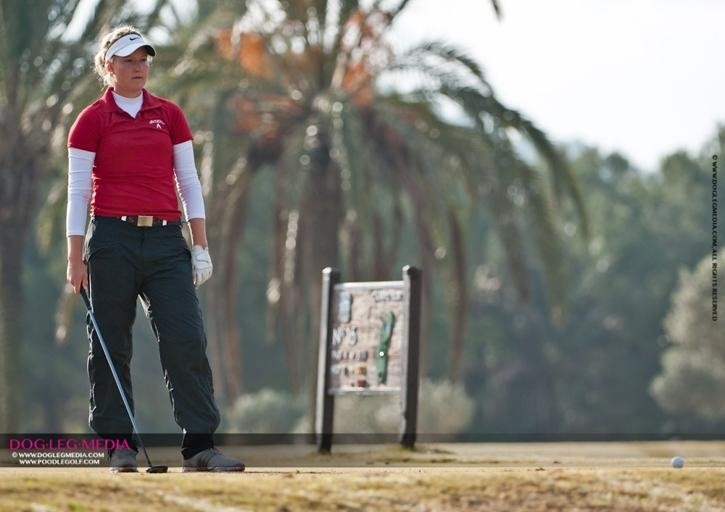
[103, 32, 158, 64]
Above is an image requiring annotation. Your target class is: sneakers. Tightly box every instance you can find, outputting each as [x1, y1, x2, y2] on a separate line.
[105, 443, 140, 473]
[179, 446, 246, 473]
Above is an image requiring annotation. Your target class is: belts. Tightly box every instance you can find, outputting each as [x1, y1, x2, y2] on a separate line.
[118, 214, 168, 227]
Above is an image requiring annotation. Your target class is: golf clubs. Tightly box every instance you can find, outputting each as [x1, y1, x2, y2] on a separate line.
[79, 283, 167, 473]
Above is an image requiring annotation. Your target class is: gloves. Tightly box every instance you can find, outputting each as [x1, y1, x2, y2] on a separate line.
[189, 243, 214, 291]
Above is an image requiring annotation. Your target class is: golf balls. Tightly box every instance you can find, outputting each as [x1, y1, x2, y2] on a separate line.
[672, 456, 684, 468]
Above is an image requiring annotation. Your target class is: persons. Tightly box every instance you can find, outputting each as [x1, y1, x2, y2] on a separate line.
[66, 27, 247, 473]
